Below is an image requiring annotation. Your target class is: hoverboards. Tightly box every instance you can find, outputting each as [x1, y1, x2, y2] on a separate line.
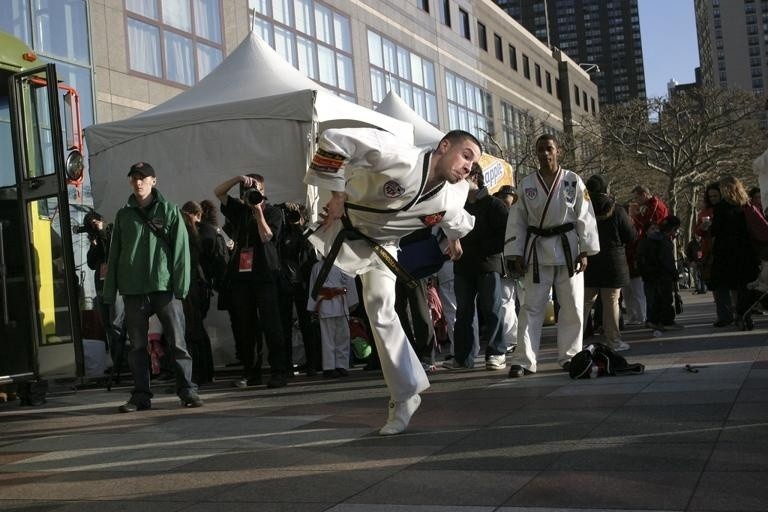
[689, 256, 709, 296]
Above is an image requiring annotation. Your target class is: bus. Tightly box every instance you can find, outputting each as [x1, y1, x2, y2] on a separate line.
[0, 25, 94, 414]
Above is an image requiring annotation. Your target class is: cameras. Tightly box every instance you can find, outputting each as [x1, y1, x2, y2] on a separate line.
[286, 211, 301, 224]
[245, 181, 267, 204]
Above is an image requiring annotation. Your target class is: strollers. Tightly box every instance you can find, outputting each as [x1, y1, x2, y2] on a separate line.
[729, 247, 768, 330]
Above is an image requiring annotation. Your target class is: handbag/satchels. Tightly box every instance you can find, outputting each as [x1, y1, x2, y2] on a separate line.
[205, 233, 229, 279]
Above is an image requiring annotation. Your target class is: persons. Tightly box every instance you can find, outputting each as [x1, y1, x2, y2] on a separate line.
[393, 162, 516, 375]
[180, 169, 383, 391]
[582, 175, 768, 351]
[84, 208, 133, 378]
[298, 125, 484, 435]
[101, 162, 202, 413]
[502, 132, 601, 378]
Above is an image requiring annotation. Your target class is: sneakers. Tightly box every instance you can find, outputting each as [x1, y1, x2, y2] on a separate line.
[485, 355, 505, 370]
[232, 375, 262, 387]
[509, 366, 524, 379]
[118, 399, 152, 413]
[182, 391, 204, 407]
[267, 373, 288, 389]
[443, 359, 462, 369]
[379, 393, 422, 435]
[614, 342, 630, 352]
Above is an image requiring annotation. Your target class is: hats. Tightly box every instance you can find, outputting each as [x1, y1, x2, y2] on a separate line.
[127, 162, 154, 178]
[492, 185, 518, 205]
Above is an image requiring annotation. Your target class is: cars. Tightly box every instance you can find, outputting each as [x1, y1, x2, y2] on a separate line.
[675, 265, 689, 289]
